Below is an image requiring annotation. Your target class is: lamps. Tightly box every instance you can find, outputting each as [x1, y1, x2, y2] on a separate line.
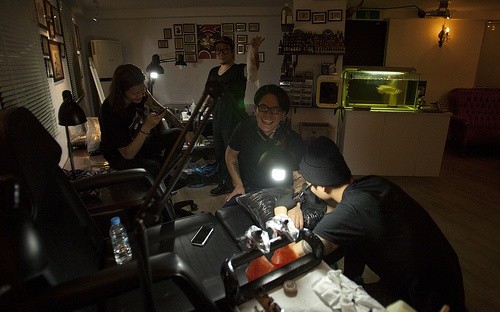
[58, 90, 87, 179]
[438, 24, 450, 49]
[146, 55, 176, 74]
[175, 54, 187, 69]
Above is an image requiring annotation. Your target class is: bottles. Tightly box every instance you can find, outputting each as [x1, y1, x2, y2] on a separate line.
[191, 100, 196, 115]
[110, 217, 132, 264]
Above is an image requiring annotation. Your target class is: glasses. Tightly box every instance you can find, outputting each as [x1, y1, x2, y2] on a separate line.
[257, 104, 281, 114]
[301, 184, 315, 193]
[216, 48, 232, 55]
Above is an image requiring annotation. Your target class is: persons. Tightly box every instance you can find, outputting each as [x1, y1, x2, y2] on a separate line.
[223, 84, 306, 229]
[201, 35, 264, 197]
[98, 64, 193, 190]
[274, 136, 466, 312]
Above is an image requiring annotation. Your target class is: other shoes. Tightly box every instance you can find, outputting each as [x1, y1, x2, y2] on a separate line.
[162, 175, 190, 193]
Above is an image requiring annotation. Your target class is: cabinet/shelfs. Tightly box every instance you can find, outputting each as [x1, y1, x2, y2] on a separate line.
[275, 51, 338, 110]
[175, 113, 215, 150]
[337, 107, 450, 177]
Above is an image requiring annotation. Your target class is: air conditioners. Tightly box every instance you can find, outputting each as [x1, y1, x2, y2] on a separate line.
[88, 40, 122, 108]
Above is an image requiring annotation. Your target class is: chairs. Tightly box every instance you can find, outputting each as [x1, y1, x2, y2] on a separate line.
[0, 105, 176, 253]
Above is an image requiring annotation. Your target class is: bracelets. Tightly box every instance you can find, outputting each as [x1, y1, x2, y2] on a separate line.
[140, 130, 149, 134]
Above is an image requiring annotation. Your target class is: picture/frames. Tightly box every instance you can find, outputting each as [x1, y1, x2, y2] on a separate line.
[311, 11, 326, 24]
[327, 10, 343, 22]
[296, 9, 311, 21]
[221, 23, 264, 63]
[157, 23, 197, 63]
[34, 0, 67, 82]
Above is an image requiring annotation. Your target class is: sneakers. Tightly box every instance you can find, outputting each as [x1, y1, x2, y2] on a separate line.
[210, 183, 233, 196]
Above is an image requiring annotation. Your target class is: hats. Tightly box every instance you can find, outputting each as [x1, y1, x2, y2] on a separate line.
[299, 136, 352, 184]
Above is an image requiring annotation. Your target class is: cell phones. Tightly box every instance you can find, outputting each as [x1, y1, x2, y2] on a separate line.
[155, 107, 168, 116]
[191, 226, 213, 246]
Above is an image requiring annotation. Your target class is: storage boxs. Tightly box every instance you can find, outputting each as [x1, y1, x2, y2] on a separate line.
[342, 71, 420, 109]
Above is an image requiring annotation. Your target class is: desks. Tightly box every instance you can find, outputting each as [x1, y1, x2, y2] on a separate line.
[175, 185, 384, 312]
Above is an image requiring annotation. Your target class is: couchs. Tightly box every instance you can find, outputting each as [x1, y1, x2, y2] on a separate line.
[448, 88, 500, 147]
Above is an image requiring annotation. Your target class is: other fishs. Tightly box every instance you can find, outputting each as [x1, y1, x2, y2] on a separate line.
[376, 84, 402, 96]
